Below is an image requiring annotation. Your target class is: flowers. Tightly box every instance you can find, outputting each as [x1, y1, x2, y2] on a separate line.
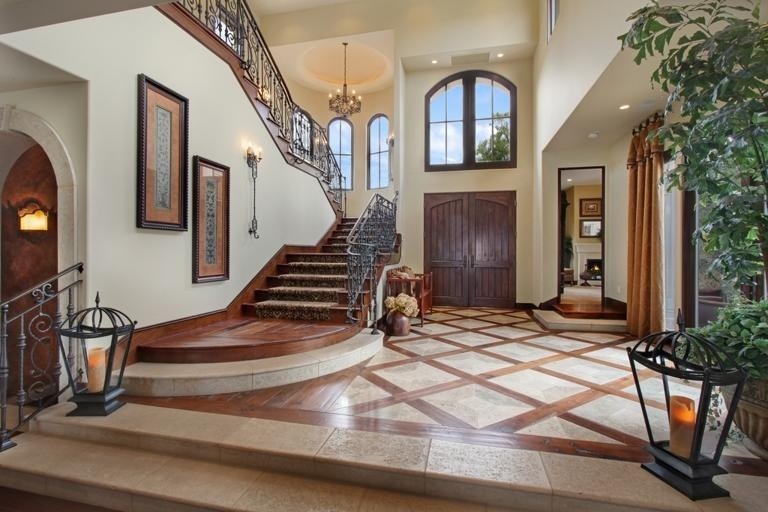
[384, 292, 420, 318]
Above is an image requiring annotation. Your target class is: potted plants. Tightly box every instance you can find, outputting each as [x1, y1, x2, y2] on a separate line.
[613, 0, 768, 471]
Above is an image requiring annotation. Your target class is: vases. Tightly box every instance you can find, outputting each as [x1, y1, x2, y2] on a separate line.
[385, 309, 410, 337]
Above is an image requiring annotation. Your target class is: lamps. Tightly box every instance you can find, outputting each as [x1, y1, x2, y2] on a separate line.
[16, 200, 49, 232]
[53, 289, 138, 419]
[246, 138, 263, 239]
[327, 42, 363, 118]
[624, 307, 747, 503]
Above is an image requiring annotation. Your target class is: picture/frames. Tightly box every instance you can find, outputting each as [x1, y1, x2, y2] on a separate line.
[578, 197, 604, 239]
[134, 70, 232, 286]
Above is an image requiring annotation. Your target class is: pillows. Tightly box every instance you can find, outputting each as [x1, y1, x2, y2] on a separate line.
[392, 265, 416, 296]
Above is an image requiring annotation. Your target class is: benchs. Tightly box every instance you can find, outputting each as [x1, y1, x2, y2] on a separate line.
[384, 268, 433, 328]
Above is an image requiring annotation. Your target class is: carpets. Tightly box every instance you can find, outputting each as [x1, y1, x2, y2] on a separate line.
[254, 216, 382, 322]
